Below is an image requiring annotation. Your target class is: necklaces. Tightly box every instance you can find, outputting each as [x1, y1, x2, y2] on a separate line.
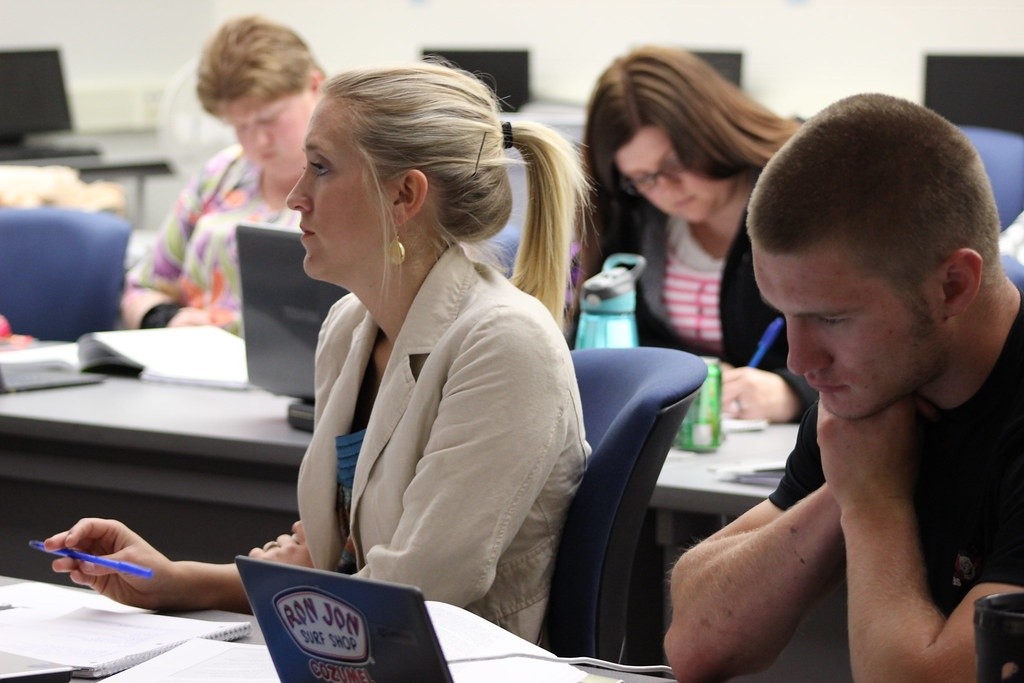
[662, 94, 1024, 682]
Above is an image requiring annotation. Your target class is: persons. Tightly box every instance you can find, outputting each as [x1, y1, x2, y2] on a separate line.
[122, 18, 340, 341]
[579, 46, 819, 425]
[46, 64, 591, 645]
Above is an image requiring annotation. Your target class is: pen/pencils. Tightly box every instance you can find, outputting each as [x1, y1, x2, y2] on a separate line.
[29, 540, 152, 578]
[749, 318, 784, 367]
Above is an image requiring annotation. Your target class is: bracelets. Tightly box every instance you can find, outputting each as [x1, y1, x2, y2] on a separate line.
[140, 303, 185, 328]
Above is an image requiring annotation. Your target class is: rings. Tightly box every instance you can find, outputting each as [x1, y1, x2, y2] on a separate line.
[733, 398, 744, 414]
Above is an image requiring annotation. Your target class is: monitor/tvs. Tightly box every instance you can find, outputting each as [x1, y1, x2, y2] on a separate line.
[422, 49, 530, 112]
[694, 52, 742, 86]
[0, 50, 72, 147]
[923, 55, 1024, 138]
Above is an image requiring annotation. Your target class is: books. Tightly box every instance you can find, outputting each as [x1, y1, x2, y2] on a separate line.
[5, 607, 252, 678]
[0, 325, 249, 389]
[97, 636, 279, 683]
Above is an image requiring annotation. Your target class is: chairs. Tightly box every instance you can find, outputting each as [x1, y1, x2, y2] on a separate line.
[0, 209, 129, 339]
[962, 122, 1023, 233]
[546, 347, 707, 671]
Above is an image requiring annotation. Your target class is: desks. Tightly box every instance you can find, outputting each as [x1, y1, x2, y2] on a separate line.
[1, 576, 677, 683]
[1, 338, 799, 631]
[0, 154, 175, 232]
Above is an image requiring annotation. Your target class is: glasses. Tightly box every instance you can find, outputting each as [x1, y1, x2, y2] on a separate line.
[620, 150, 689, 195]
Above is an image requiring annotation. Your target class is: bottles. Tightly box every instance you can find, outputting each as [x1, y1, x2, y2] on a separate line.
[574, 253, 647, 349]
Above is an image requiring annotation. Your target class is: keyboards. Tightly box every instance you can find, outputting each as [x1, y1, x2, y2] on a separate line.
[0, 146, 100, 162]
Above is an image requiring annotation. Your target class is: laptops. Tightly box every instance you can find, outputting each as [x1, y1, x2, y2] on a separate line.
[0, 650, 72, 683]
[234, 555, 455, 683]
[236, 226, 354, 401]
